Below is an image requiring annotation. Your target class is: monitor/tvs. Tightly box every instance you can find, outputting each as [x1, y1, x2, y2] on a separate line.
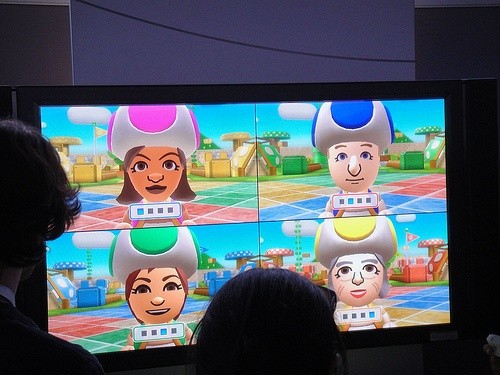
[2, 79, 462, 370]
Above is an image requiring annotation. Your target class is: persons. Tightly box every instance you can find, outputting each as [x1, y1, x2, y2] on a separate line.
[185, 267, 351, 374]
[0, 120, 107, 375]
